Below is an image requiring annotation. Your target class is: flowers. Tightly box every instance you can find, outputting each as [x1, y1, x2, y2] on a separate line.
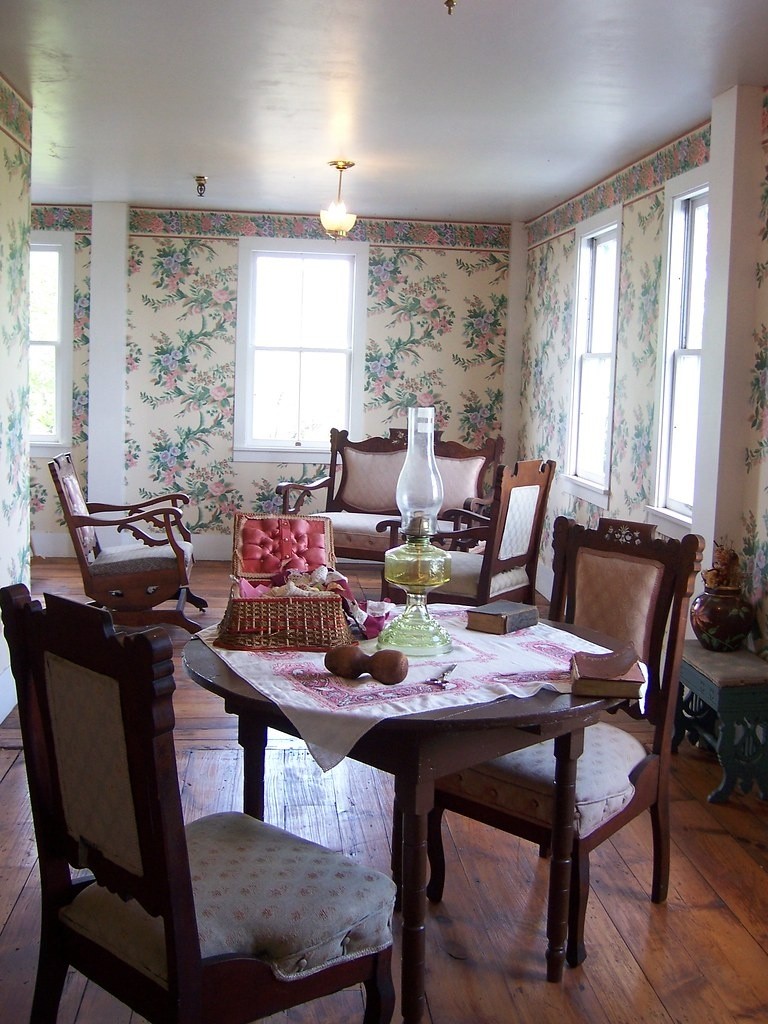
[701, 545, 747, 590]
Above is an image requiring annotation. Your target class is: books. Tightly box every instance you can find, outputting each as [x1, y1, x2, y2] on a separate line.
[569, 641, 645, 699]
[466, 600, 539, 634]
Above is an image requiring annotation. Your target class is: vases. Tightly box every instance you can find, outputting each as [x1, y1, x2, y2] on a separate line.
[690, 588, 754, 651]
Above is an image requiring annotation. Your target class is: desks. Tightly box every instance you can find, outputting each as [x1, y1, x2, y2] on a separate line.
[184, 604, 640, 1024]
[670, 640, 768, 803]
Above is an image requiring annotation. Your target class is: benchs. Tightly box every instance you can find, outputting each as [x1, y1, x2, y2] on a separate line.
[277, 428, 493, 562]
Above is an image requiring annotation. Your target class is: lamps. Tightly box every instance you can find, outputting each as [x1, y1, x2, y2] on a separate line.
[375, 405, 452, 656]
[320, 160, 357, 242]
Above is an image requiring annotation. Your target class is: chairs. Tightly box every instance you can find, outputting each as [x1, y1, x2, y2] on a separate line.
[48, 452, 208, 634]
[232, 513, 333, 588]
[376, 460, 556, 604]
[426, 516, 705, 968]
[0, 583, 397, 1024]
